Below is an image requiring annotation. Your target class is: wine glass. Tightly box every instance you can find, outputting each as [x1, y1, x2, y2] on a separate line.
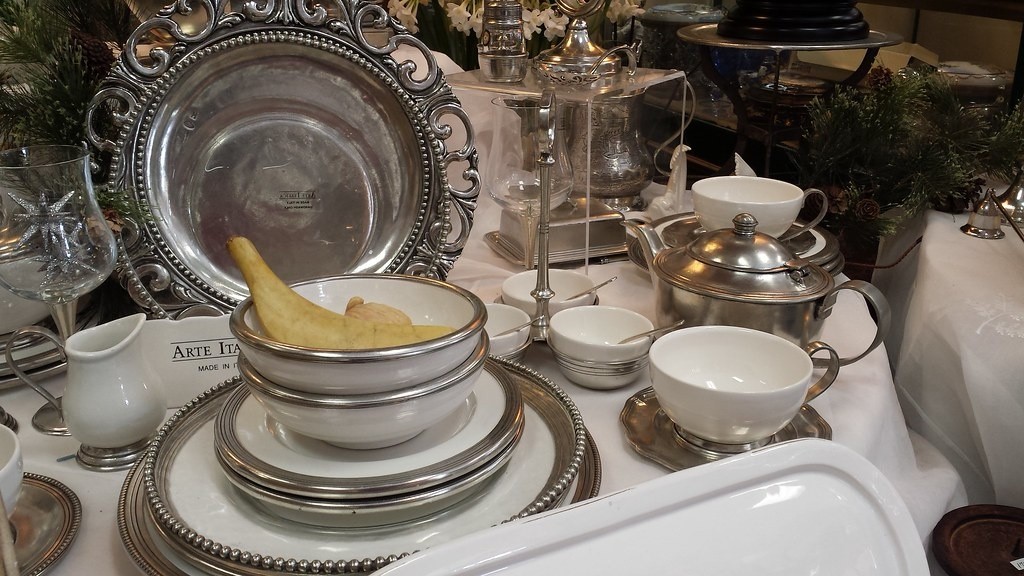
[486, 97, 574, 269]
[0, 146, 119, 343]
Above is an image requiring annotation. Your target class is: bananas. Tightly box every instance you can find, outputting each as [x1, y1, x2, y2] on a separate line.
[225, 235, 455, 349]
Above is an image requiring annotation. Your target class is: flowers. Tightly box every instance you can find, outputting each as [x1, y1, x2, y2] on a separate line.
[386, 0, 645, 43]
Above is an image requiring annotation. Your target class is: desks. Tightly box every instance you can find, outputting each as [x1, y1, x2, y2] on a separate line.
[0, 271, 932, 576]
[870, 179, 1022, 508]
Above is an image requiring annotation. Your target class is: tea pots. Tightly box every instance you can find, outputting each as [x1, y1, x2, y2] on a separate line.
[620, 219, 892, 369]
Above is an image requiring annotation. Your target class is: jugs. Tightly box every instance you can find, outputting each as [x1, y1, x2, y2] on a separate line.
[558, 72, 706, 220]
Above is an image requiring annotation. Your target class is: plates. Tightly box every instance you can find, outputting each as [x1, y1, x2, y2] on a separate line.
[145, 356, 587, 576]
[620, 386, 833, 472]
[214, 361, 527, 535]
[628, 212, 846, 277]
[0, 301, 108, 389]
[0, 473, 82, 575]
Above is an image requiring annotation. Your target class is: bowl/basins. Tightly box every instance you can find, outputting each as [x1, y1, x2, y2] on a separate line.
[229, 273, 491, 449]
[548, 305, 654, 362]
[502, 269, 597, 317]
[485, 303, 531, 356]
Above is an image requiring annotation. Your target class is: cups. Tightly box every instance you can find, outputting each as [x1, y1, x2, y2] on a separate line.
[0, 424, 24, 517]
[692, 176, 829, 242]
[650, 325, 840, 453]
[6, 313, 169, 472]
